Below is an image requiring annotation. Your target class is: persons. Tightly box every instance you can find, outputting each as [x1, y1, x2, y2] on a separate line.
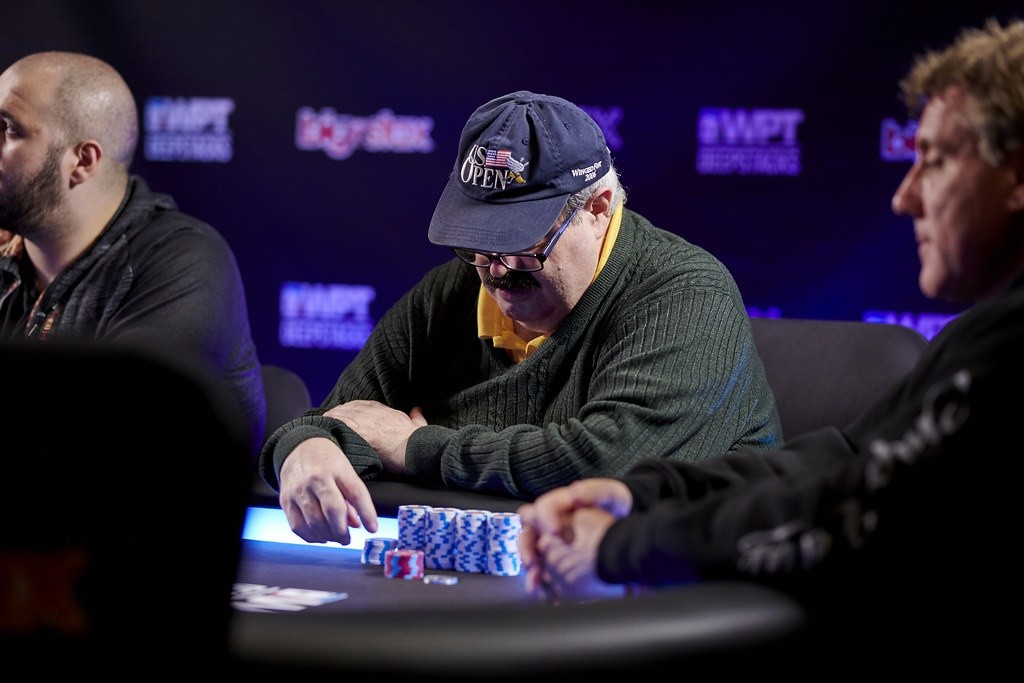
[0, 336, 252, 683]
[514, 9, 1023, 683]
[258, 91, 785, 547]
[0, 51, 266, 448]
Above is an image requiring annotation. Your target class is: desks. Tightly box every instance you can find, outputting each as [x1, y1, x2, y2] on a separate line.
[234, 477, 811, 683]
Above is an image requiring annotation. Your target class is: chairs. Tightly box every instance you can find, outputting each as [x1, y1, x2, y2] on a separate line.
[0, 331, 250, 683]
[751, 317, 924, 432]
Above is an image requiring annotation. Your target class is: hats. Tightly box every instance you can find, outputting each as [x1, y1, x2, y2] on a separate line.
[427, 90, 610, 254]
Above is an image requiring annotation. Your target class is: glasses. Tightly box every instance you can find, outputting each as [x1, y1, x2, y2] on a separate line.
[454, 197, 582, 272]
